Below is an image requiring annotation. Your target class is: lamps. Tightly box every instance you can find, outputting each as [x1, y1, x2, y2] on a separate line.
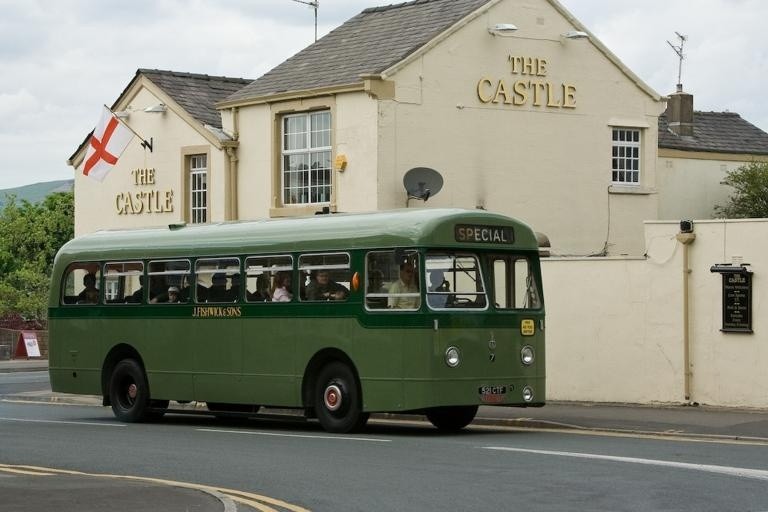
[486, 23, 589, 41]
[111, 103, 168, 117]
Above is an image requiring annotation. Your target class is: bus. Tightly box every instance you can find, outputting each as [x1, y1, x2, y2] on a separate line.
[48, 206, 546, 433]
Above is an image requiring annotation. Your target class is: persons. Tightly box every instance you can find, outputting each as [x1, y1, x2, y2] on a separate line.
[368, 260, 456, 310]
[77, 273, 100, 303]
[132, 271, 293, 303]
[305, 270, 349, 300]
